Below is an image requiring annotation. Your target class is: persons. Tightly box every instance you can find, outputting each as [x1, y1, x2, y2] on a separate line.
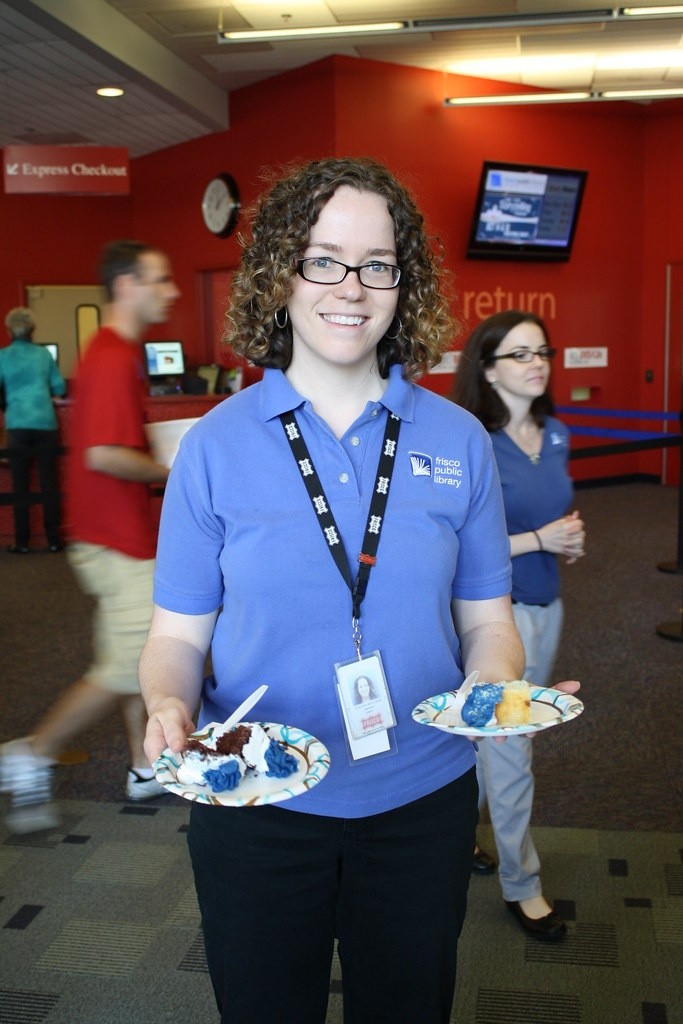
[0, 241, 181, 833]
[137, 158, 580, 1024]
[448, 309, 585, 944]
[353, 675, 376, 705]
[0, 307, 68, 556]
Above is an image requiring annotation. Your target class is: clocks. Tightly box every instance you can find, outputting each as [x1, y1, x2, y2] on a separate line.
[200, 172, 241, 239]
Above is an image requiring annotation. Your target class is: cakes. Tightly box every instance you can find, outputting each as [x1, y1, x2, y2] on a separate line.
[458, 679, 533, 729]
[175, 721, 300, 792]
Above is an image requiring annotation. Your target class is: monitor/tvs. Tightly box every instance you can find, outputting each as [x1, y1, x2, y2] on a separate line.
[143, 341, 185, 377]
[465, 160, 588, 262]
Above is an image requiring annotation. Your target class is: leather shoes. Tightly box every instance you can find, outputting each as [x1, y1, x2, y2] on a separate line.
[503, 897, 567, 943]
[469, 849, 496, 875]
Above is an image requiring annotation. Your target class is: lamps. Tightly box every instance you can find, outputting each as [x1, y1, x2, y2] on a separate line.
[217, 5, 683, 44]
[442, 87, 683, 107]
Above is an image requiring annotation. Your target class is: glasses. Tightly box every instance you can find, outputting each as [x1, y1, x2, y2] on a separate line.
[489, 347, 555, 365]
[288, 257, 402, 291]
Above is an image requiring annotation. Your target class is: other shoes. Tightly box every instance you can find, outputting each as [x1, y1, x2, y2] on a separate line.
[7, 543, 29, 553]
[49, 543, 62, 552]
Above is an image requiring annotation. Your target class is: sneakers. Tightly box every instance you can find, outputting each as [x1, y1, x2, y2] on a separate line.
[125, 768, 170, 800]
[0, 738, 59, 834]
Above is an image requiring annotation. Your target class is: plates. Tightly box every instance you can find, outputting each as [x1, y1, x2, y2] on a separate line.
[411, 683, 585, 735]
[152, 721, 331, 808]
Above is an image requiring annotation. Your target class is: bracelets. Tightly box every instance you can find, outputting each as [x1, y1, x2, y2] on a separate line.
[533, 530, 543, 551]
